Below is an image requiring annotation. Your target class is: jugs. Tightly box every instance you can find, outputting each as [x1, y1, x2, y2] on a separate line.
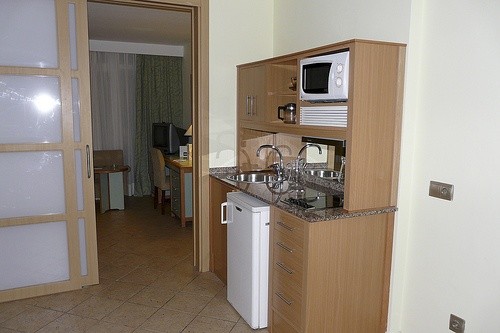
[277, 103, 296, 124]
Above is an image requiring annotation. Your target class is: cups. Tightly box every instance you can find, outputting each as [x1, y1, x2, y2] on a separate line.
[246, 95, 257, 117]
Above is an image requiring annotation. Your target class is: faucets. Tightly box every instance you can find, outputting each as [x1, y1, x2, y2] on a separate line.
[297, 143, 322, 161]
[256, 145, 284, 172]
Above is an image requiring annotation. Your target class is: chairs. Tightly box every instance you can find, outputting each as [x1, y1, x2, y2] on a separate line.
[149, 148, 170, 214]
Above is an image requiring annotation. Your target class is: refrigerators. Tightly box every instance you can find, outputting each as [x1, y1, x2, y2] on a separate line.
[225, 191, 270, 330]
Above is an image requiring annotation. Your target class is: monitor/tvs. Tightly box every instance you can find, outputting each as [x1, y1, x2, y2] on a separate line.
[151, 123, 189, 154]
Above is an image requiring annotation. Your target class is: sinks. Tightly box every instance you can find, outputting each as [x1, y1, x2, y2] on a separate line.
[225, 171, 280, 184]
[304, 169, 343, 178]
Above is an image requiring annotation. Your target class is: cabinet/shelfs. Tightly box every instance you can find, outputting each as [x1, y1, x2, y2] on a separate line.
[209, 175, 232, 286]
[267, 205, 395, 333]
[164, 154, 193, 228]
[236, 39, 407, 211]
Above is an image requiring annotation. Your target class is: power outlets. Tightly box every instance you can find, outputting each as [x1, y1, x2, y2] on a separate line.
[448, 314, 465, 333]
[428, 180, 453, 201]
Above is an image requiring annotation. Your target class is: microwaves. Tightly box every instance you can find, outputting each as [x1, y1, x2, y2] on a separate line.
[299, 51, 350, 103]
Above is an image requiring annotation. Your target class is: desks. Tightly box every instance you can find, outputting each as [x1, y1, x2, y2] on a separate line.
[94, 166, 131, 215]
